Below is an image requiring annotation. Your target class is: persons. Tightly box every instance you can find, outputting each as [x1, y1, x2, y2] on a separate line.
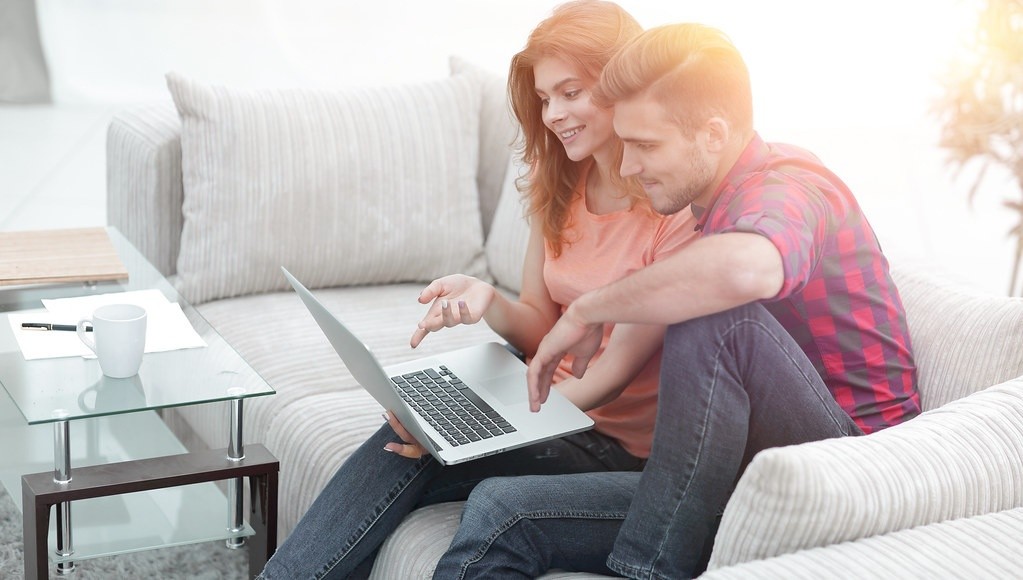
[254, 0, 701, 580]
[431, 23, 919, 580]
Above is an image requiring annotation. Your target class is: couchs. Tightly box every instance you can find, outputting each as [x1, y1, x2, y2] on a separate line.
[105, 104, 1023, 580]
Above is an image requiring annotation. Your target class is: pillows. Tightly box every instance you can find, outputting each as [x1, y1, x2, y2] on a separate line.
[886, 258, 1023, 412]
[443, 53, 518, 246]
[163, 71, 495, 310]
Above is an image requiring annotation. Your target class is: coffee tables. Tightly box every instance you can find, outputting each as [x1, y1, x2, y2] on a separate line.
[0, 226, 280, 580]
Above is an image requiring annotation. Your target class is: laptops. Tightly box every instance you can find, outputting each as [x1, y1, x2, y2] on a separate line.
[279, 266, 596, 466]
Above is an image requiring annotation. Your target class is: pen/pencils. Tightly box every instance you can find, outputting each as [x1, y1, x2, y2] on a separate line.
[21, 322, 93, 332]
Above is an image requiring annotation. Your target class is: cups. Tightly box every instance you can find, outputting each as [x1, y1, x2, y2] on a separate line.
[76, 303, 146, 378]
